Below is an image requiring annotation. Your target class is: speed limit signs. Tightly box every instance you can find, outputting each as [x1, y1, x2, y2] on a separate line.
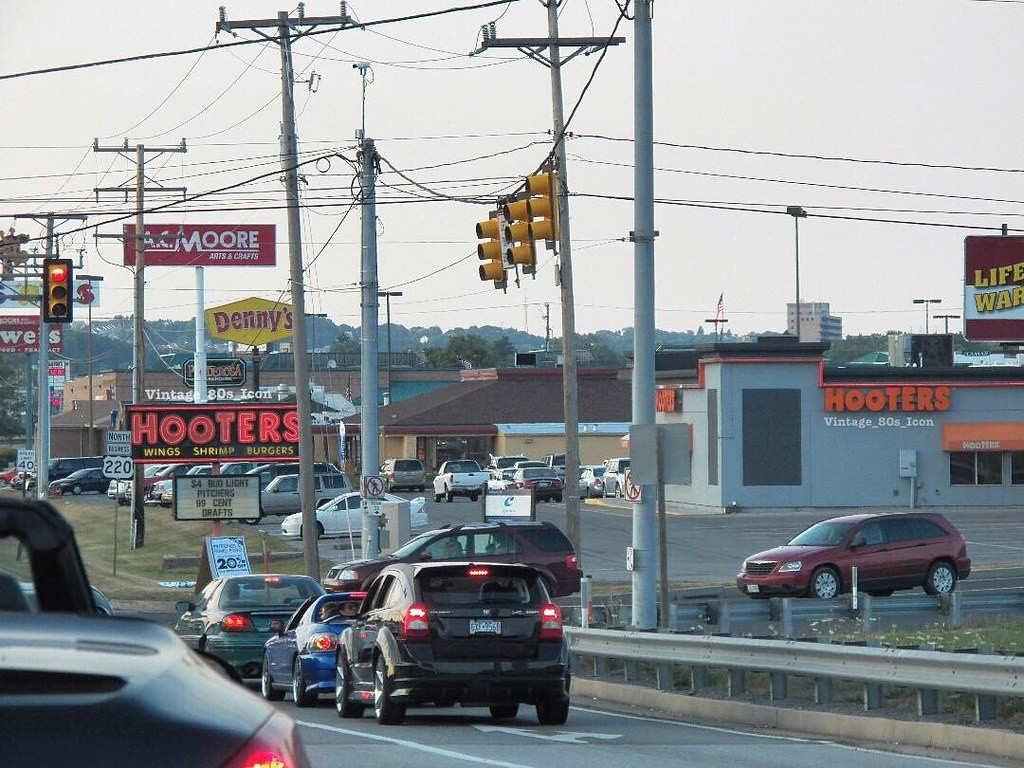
[18, 451, 34, 472]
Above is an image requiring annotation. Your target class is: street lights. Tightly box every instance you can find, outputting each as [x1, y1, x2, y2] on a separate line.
[705, 319, 727, 343]
[912, 299, 942, 334]
[787, 206, 807, 340]
[305, 314, 328, 372]
[933, 315, 961, 335]
[378, 290, 402, 404]
[76, 274, 103, 456]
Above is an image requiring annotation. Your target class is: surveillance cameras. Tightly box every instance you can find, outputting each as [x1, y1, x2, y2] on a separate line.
[352, 61, 370, 69]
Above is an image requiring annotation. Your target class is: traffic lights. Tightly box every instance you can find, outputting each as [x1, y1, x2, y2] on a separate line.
[475, 213, 508, 289]
[524, 172, 560, 243]
[503, 192, 538, 267]
[43, 258, 74, 324]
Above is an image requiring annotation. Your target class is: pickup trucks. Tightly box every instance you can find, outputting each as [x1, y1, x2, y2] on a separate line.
[432, 460, 491, 503]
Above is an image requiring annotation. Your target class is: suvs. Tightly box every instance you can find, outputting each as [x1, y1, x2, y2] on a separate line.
[736, 512, 972, 598]
[173, 563, 569, 726]
[488, 454, 630, 503]
[380, 460, 425, 492]
[325, 520, 582, 600]
[108, 462, 353, 526]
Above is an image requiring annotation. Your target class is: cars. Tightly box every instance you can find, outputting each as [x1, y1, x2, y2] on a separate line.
[0, 499, 309, 768]
[282, 491, 429, 540]
[0, 457, 111, 497]
